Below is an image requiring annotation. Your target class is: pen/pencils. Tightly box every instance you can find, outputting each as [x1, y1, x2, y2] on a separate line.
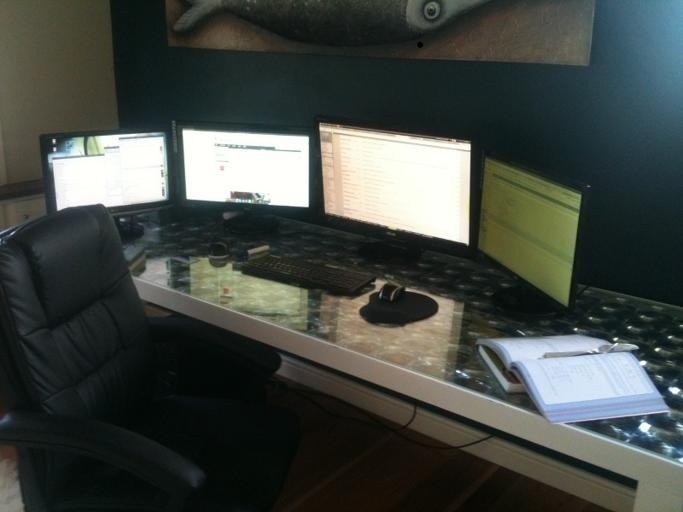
[246, 245, 270, 254]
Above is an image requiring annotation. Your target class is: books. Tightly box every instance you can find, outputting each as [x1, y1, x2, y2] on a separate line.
[475, 334, 670, 424]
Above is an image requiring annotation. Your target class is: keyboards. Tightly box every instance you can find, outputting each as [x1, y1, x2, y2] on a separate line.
[241, 254, 377, 294]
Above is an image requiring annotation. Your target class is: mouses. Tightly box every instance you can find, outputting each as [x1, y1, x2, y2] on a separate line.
[378, 281, 404, 301]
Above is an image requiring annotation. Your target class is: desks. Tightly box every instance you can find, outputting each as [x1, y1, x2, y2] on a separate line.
[0, 194, 682, 510]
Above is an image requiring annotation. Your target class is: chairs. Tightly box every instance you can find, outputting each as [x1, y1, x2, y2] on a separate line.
[0, 208, 297, 510]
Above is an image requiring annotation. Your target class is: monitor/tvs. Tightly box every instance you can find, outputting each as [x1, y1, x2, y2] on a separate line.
[38, 125, 174, 239]
[311, 113, 476, 279]
[168, 120, 316, 235]
[474, 147, 591, 320]
